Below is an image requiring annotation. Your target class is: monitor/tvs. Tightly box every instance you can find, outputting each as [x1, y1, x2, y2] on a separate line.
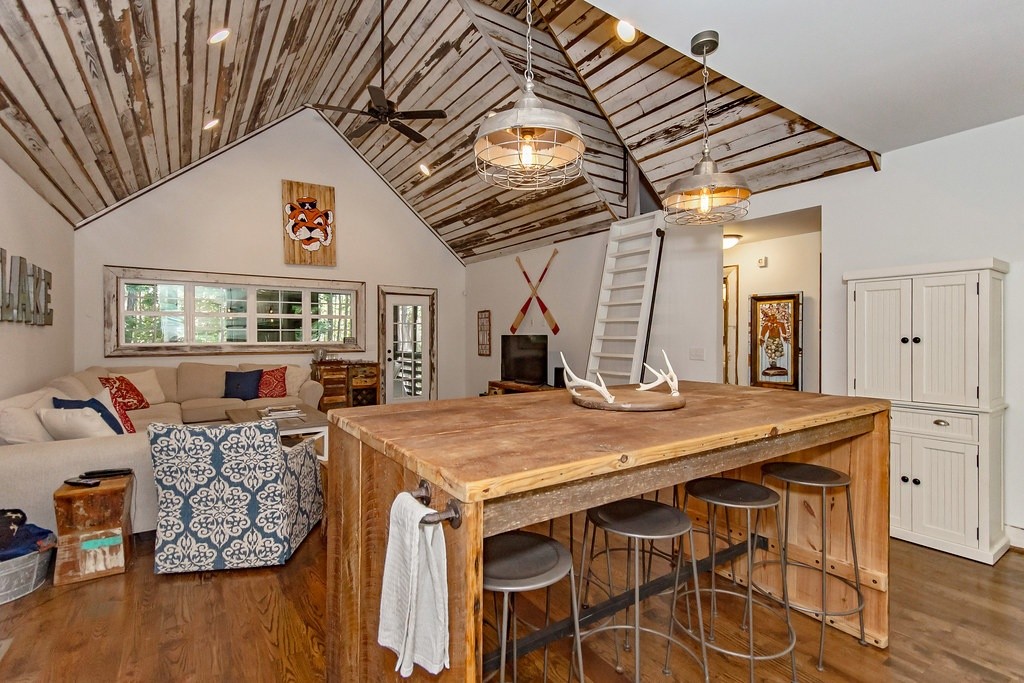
[501, 334, 554, 388]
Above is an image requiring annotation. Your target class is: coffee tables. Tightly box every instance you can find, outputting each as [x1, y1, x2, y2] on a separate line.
[225, 403, 329, 462]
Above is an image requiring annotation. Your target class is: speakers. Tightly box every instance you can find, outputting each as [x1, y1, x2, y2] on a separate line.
[554, 367, 566, 389]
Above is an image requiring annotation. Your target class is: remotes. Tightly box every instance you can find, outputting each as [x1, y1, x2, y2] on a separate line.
[64, 477, 101, 487]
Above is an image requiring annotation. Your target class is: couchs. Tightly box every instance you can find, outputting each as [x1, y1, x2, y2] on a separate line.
[176, 361, 324, 423]
[0, 365, 182, 541]
[145, 420, 325, 575]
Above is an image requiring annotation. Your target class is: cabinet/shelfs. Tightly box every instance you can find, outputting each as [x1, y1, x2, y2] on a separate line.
[840, 257, 1010, 567]
[488, 378, 566, 396]
[309, 359, 380, 414]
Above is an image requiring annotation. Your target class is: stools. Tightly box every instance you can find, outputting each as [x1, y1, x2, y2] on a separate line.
[480, 461, 867, 683]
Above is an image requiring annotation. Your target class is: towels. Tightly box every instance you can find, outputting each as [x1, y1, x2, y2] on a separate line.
[0, 508, 28, 549]
[377, 491, 451, 678]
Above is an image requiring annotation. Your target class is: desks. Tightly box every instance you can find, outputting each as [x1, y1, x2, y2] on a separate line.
[324, 380, 893, 683]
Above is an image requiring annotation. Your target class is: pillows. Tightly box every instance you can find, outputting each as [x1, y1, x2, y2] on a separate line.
[52, 396, 124, 434]
[36, 406, 117, 440]
[98, 376, 150, 410]
[224, 370, 263, 400]
[257, 366, 288, 398]
[281, 364, 312, 395]
[82, 387, 128, 434]
[109, 369, 166, 404]
[108, 390, 137, 433]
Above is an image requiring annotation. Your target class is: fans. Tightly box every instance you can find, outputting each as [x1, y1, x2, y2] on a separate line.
[311, 0, 447, 143]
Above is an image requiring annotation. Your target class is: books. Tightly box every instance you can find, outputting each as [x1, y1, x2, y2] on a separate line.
[257, 403, 307, 420]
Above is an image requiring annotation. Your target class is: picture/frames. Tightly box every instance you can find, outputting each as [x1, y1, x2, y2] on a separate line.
[746, 291, 804, 393]
[477, 310, 491, 356]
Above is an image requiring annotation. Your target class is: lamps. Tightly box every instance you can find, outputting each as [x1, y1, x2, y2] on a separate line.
[660, 30, 752, 225]
[472, 0, 586, 193]
